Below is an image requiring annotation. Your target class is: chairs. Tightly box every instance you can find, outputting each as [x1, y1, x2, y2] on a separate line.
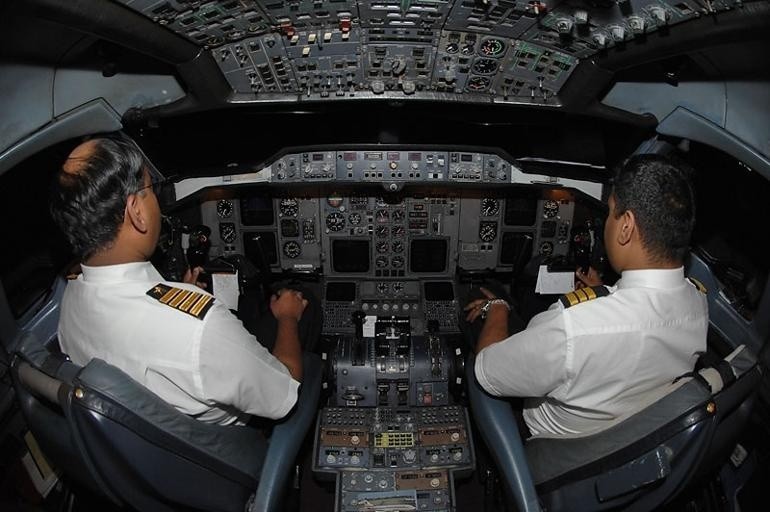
[462, 338, 769, 509]
[0, 321, 326, 511]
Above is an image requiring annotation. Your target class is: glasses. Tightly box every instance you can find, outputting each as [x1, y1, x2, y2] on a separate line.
[137, 177, 161, 193]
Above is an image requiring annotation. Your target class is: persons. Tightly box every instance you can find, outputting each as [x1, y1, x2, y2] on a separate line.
[462, 163, 710, 472]
[50, 137, 318, 442]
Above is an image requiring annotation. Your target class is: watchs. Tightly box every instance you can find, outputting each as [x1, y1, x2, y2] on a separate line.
[479, 297, 512, 319]
